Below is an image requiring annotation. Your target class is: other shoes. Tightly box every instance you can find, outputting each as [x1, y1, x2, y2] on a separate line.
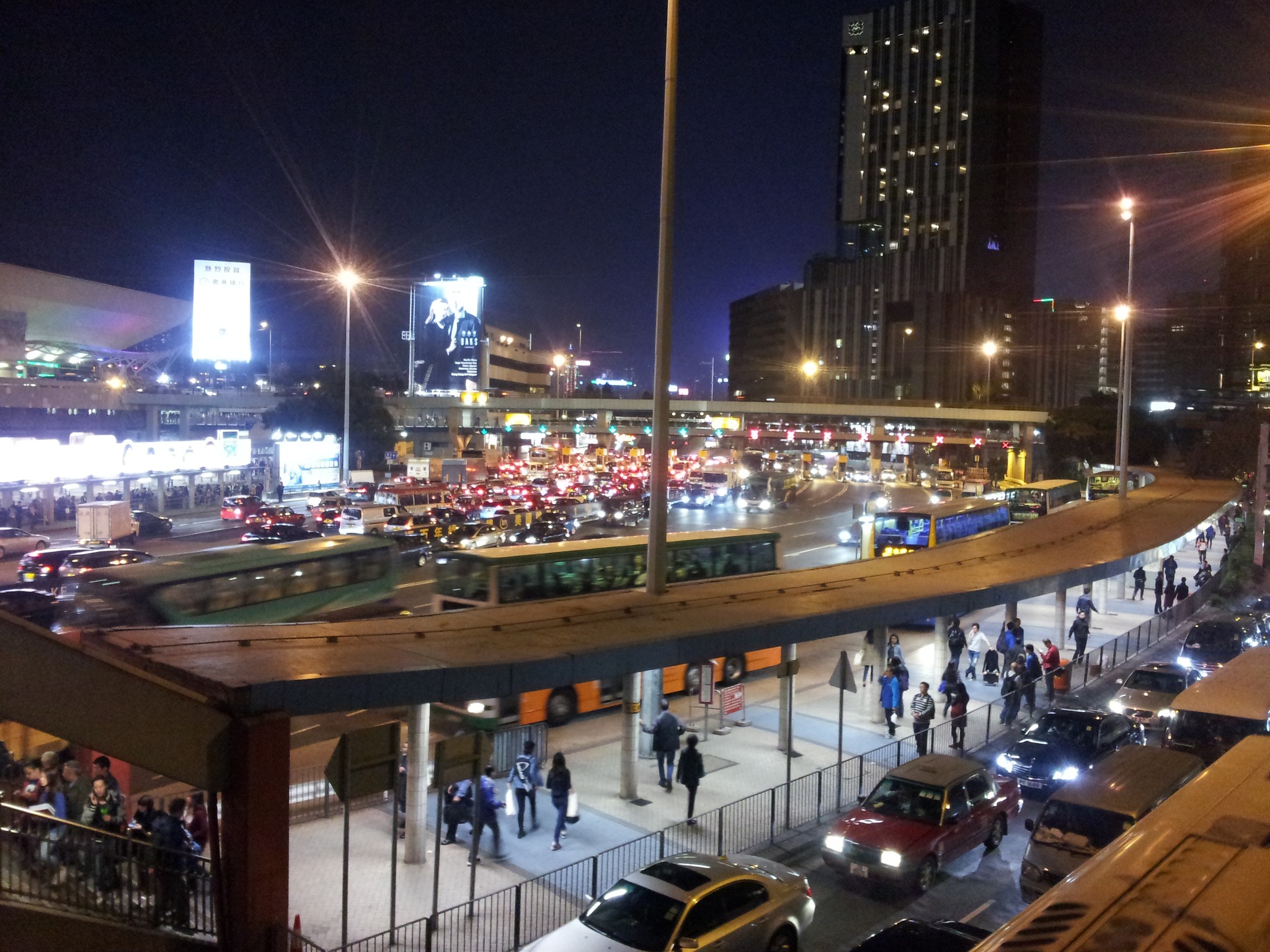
[517, 831, 526, 838]
[965, 671, 968, 677]
[400, 821, 406, 829]
[468, 858, 480, 866]
[958, 741, 962, 748]
[688, 820, 696, 825]
[666, 783, 672, 791]
[885, 733, 894, 738]
[400, 833, 405, 838]
[949, 743, 958, 749]
[971, 676, 976, 679]
[552, 843, 560, 851]
[561, 831, 567, 838]
[441, 839, 454, 845]
[659, 780, 666, 787]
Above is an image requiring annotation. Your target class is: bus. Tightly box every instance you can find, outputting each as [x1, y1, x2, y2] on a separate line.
[867, 472, 1140, 632]
[429, 527, 781, 728]
[75, 539, 396, 628]
[0, 501, 174, 621]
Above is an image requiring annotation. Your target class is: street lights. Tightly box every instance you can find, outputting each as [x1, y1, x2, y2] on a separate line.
[1115, 192, 1134, 507]
[980, 337, 996, 410]
[259, 319, 273, 398]
[551, 353, 564, 422]
[1251, 341, 1265, 389]
[339, 266, 360, 486]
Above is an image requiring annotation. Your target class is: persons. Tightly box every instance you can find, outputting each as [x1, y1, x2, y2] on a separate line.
[249, 457, 273, 476]
[251, 483, 264, 497]
[95, 490, 121, 501]
[1196, 525, 1216, 564]
[397, 741, 408, 839]
[130, 487, 154, 511]
[53, 492, 87, 521]
[317, 480, 321, 490]
[163, 486, 189, 509]
[1217, 505, 1245, 569]
[1068, 587, 1099, 669]
[441, 741, 572, 866]
[276, 482, 285, 503]
[0, 496, 44, 532]
[194, 483, 221, 505]
[861, 616, 1059, 757]
[1131, 555, 1212, 614]
[223, 481, 249, 497]
[417, 289, 482, 396]
[523, 544, 762, 595]
[162, 412, 180, 425]
[640, 699, 705, 825]
[0, 741, 208, 929]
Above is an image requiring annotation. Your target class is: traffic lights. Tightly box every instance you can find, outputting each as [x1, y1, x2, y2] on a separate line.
[480, 427, 488, 435]
[504, 424, 513, 432]
[748, 430, 759, 440]
[897, 435, 906, 443]
[573, 424, 582, 434]
[714, 428, 723, 436]
[1002, 443, 1010, 449]
[973, 437, 982, 446]
[609, 425, 617, 433]
[537, 424, 547, 433]
[784, 431, 796, 441]
[859, 433, 871, 441]
[934, 434, 945, 445]
[644, 427, 652, 434]
[821, 431, 833, 439]
[679, 428, 688, 436]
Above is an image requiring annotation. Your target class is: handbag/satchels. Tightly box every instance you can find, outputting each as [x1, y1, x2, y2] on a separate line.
[853, 649, 864, 665]
[505, 788, 517, 815]
[566, 793, 578, 823]
[891, 710, 897, 724]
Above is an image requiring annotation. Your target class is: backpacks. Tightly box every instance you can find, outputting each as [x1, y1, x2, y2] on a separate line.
[513, 755, 534, 792]
[1001, 670, 1018, 700]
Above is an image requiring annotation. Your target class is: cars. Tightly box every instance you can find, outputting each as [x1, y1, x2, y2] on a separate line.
[515, 850, 817, 952]
[219, 444, 800, 572]
[811, 465, 933, 484]
[1168, 592, 1270, 759]
[821, 662, 1204, 891]
[849, 919, 994, 952]
[840, 521, 880, 545]
